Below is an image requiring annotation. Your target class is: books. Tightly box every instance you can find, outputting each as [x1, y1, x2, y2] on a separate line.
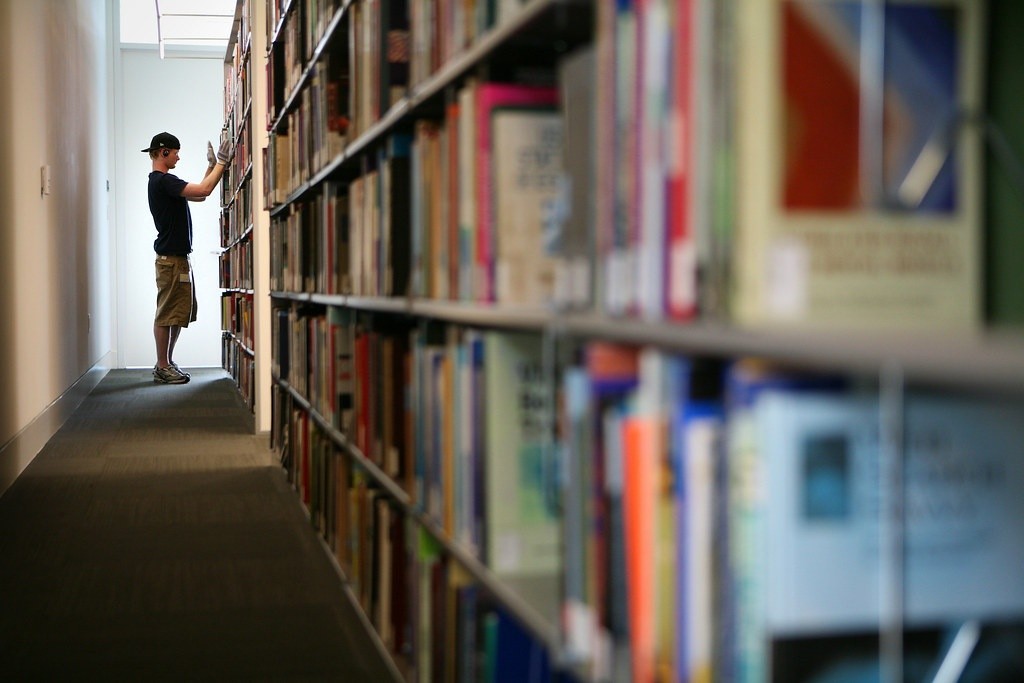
[216, 0, 1024, 683]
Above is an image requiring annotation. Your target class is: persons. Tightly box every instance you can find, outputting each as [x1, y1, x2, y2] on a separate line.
[141, 132, 231, 384]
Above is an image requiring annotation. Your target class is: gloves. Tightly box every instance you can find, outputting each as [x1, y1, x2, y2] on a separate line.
[217, 139, 233, 164]
[207, 141, 216, 167]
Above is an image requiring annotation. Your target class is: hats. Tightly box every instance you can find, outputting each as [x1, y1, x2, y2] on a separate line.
[141, 132, 180, 152]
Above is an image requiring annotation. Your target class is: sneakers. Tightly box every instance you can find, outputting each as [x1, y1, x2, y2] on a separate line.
[154, 365, 190, 384]
[153, 362, 190, 377]
